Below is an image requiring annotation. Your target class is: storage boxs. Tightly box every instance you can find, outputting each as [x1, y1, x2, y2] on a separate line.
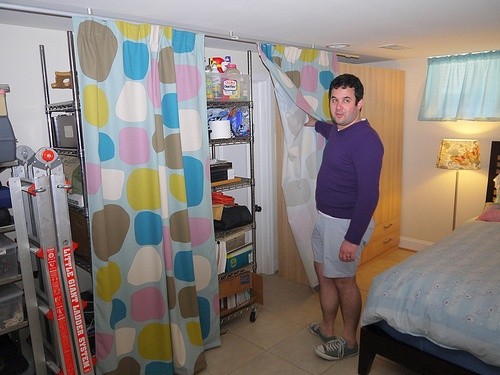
[0, 235, 18, 280]
[219, 232, 264, 318]
[0, 283, 24, 330]
[55, 115, 79, 148]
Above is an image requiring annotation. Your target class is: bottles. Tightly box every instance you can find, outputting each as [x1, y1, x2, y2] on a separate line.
[203, 56, 243, 102]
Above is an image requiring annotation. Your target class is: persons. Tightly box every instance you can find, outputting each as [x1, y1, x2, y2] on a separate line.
[304, 73, 384, 360]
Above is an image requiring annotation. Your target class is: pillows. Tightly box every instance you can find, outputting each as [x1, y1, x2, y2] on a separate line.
[476, 205, 500, 222]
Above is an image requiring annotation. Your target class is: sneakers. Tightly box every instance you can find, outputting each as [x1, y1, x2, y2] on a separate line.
[314, 336, 358, 361]
[309, 322, 337, 343]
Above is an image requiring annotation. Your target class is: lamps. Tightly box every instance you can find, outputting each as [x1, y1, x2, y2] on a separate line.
[436, 138, 481, 232]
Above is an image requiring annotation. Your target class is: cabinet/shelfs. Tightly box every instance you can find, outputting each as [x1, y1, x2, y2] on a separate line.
[277, 62, 405, 287]
[0, 160, 30, 375]
[39, 31, 258, 357]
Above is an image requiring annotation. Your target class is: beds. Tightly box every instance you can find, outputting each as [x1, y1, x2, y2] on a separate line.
[358, 141, 500, 375]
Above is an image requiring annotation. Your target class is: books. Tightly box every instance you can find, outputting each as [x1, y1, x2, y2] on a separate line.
[221, 289, 251, 310]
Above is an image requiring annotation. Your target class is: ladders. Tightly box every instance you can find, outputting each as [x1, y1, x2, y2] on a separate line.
[14, 144, 96, 374]
